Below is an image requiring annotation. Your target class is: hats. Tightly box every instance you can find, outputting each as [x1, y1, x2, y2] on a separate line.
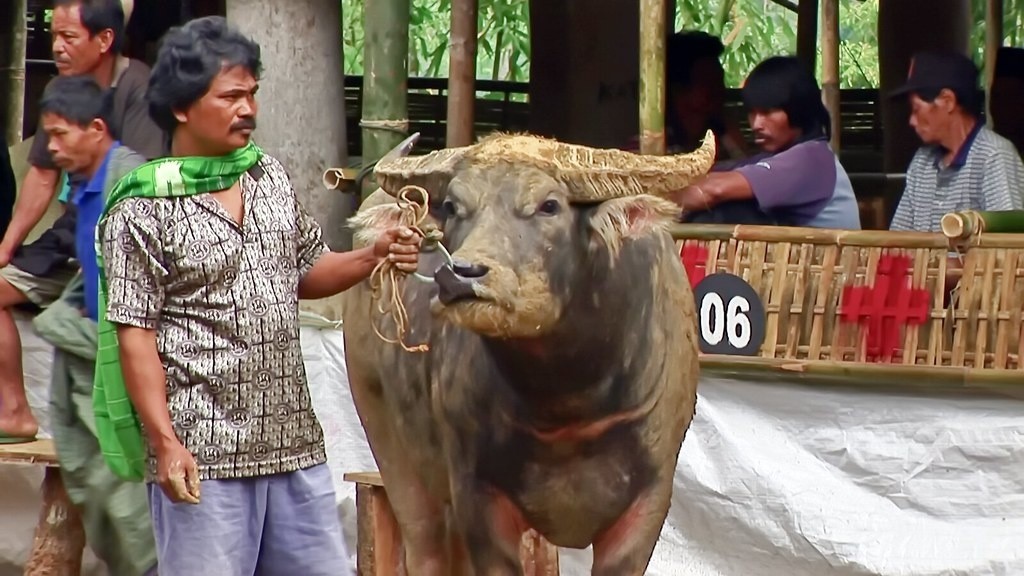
[882, 49, 979, 105]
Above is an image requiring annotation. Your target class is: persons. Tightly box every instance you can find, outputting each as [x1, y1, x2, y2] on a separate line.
[889, 50, 1024, 307]
[621, 30, 862, 231]
[0, 0, 163, 445]
[101, 16, 420, 576]
[38, 75, 150, 320]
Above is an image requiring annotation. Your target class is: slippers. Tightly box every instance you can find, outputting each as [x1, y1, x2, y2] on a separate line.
[0, 436, 36, 443]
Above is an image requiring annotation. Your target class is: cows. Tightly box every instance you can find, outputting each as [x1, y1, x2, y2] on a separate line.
[341, 128, 718, 570]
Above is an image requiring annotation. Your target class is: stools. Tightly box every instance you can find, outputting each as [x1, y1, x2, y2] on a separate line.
[0, 438, 87, 576]
[341, 471, 561, 576]
